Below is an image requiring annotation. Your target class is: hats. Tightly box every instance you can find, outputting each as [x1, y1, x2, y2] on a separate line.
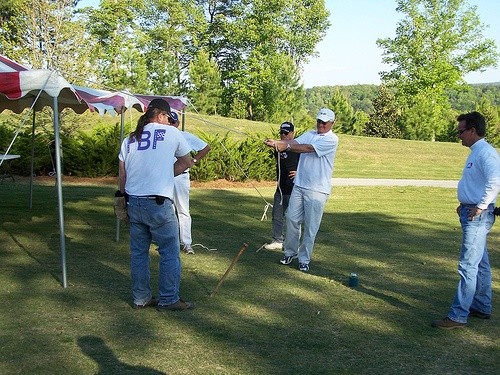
[279, 122, 294, 132]
[147, 98, 177, 121]
[316, 108, 335, 122]
[171, 112, 178, 120]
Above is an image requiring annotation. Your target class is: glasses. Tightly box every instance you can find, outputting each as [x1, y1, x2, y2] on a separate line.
[456, 128, 467, 135]
[164, 114, 171, 122]
[317, 120, 327, 125]
[279, 131, 289, 135]
[171, 120, 177, 124]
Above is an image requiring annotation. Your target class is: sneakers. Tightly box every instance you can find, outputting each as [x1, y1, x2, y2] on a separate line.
[264, 241, 284, 250]
[133, 296, 160, 309]
[183, 244, 195, 254]
[157, 299, 191, 311]
[431, 317, 464, 329]
[280, 253, 298, 264]
[299, 263, 309, 271]
[468, 307, 490, 319]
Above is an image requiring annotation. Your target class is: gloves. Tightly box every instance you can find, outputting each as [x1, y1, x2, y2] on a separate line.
[114, 189, 130, 224]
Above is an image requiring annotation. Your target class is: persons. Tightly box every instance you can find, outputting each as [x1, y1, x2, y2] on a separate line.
[432, 112, 500, 330]
[266, 122, 302, 253]
[114, 99, 210, 311]
[263, 108, 338, 271]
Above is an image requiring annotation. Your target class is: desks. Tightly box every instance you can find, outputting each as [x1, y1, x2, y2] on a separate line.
[0, 155, 20, 182]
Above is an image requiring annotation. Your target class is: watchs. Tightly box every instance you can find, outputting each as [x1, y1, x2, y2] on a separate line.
[287, 143, 291, 151]
[192, 159, 198, 163]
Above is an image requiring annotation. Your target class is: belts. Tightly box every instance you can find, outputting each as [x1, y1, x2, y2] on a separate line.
[130, 195, 157, 199]
[181, 172, 187, 174]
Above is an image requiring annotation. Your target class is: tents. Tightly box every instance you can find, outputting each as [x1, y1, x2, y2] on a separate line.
[0, 55, 192, 288]
